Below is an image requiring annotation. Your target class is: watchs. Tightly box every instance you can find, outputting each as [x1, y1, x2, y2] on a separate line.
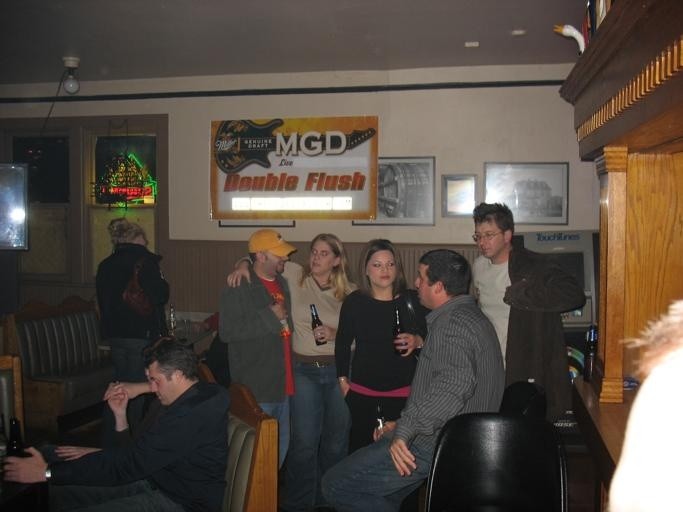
[45, 463, 52, 483]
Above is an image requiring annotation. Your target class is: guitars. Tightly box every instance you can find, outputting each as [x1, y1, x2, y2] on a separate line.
[213, 119, 376, 175]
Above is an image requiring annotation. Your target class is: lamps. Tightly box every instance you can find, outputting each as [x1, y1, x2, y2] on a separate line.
[62, 57, 81, 94]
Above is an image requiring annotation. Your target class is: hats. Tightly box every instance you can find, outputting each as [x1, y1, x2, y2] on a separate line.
[249, 229, 298, 256]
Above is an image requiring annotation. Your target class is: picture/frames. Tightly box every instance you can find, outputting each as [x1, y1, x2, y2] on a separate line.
[219, 219, 295, 228]
[484, 160, 568, 224]
[352, 155, 436, 226]
[440, 174, 477, 218]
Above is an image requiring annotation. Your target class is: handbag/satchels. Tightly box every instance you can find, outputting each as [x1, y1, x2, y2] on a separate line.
[123, 254, 157, 316]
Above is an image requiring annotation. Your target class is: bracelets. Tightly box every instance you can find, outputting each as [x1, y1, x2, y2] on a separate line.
[339, 378, 347, 380]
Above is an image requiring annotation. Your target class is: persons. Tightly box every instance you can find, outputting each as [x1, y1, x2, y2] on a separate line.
[470, 202, 586, 424]
[102, 336, 194, 440]
[226, 233, 358, 512]
[0, 345, 232, 512]
[320, 249, 506, 512]
[608, 300, 683, 512]
[335, 239, 428, 512]
[95, 217, 170, 432]
[218, 230, 298, 471]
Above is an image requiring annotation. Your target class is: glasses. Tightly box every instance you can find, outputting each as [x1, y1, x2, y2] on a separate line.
[473, 230, 506, 240]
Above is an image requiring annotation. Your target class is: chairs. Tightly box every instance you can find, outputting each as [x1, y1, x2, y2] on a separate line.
[220, 380, 280, 512]
[0, 356, 24, 442]
[499, 384, 548, 420]
[423, 412, 570, 512]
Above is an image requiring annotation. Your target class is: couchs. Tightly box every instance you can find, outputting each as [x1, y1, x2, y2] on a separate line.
[3, 298, 120, 434]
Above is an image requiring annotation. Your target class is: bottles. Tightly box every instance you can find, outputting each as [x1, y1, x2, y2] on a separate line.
[169, 304, 177, 330]
[8, 417, 23, 458]
[395, 306, 406, 355]
[376, 405, 386, 443]
[584, 326, 597, 381]
[309, 304, 328, 346]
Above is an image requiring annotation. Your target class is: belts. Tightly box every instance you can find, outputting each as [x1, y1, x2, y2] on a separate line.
[305, 360, 334, 367]
